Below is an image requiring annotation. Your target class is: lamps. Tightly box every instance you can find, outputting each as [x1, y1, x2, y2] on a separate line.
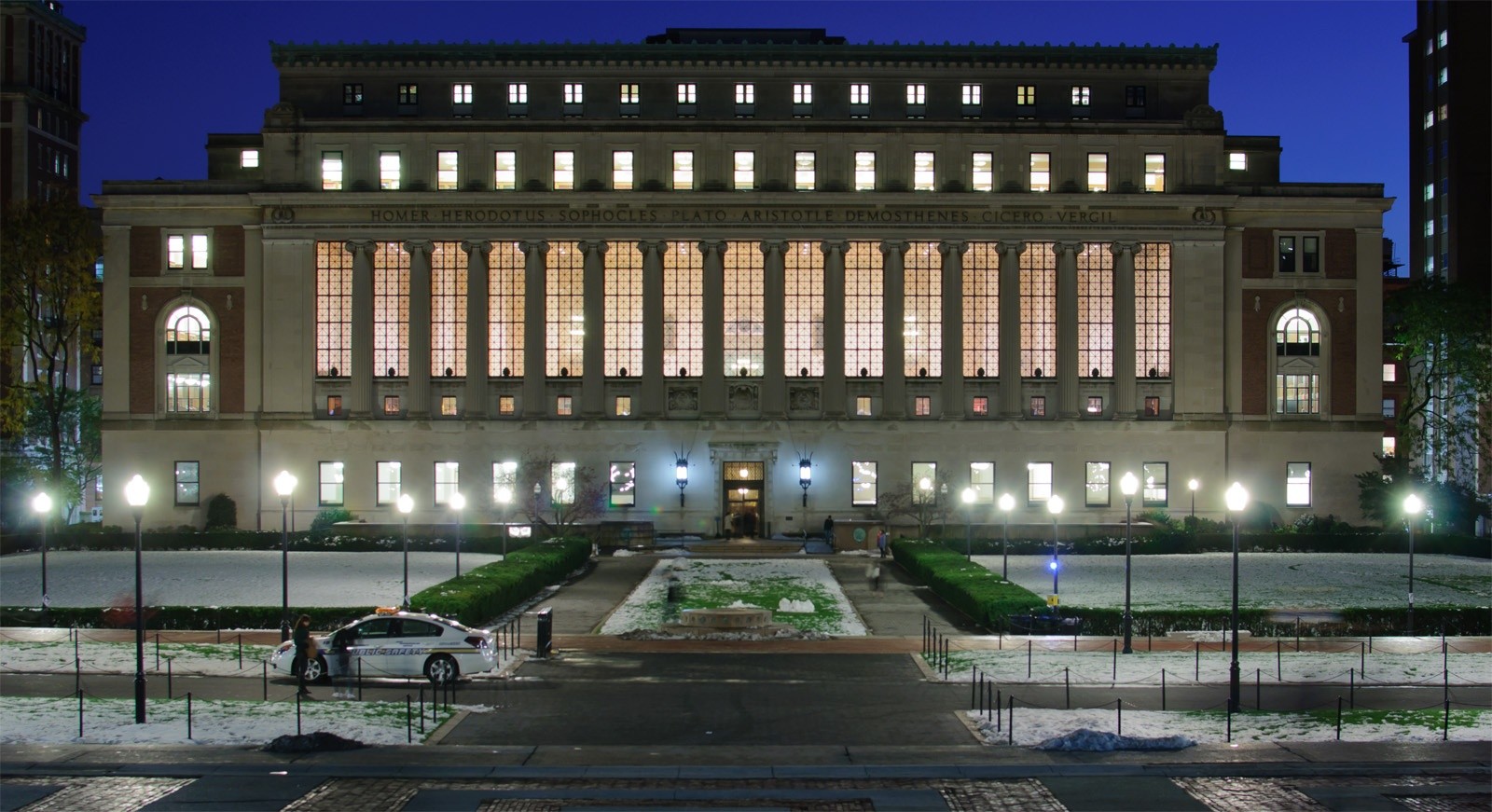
[796, 441, 814, 508]
[673, 439, 691, 506]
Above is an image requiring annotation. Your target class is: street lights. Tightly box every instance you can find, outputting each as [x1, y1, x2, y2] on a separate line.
[124, 474, 151, 723]
[1404, 495, 1424, 607]
[397, 460, 564, 607]
[669, 444, 816, 559]
[275, 471, 299, 645]
[31, 491, 61, 610]
[916, 477, 1250, 711]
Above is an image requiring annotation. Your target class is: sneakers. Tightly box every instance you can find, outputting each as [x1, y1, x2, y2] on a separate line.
[333, 691, 344, 698]
[347, 693, 354, 700]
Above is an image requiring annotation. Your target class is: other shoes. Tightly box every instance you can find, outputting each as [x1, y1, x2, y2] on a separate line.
[297, 686, 310, 694]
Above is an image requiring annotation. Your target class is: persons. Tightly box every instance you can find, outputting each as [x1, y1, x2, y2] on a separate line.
[723, 510, 732, 542]
[878, 529, 887, 558]
[732, 508, 756, 539]
[293, 614, 312, 694]
[823, 514, 833, 546]
[324, 617, 357, 700]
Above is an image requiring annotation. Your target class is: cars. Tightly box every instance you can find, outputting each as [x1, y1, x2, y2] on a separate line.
[269, 607, 499, 687]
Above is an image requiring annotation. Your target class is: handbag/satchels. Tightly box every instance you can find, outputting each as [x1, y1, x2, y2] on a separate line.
[306, 638, 318, 658]
